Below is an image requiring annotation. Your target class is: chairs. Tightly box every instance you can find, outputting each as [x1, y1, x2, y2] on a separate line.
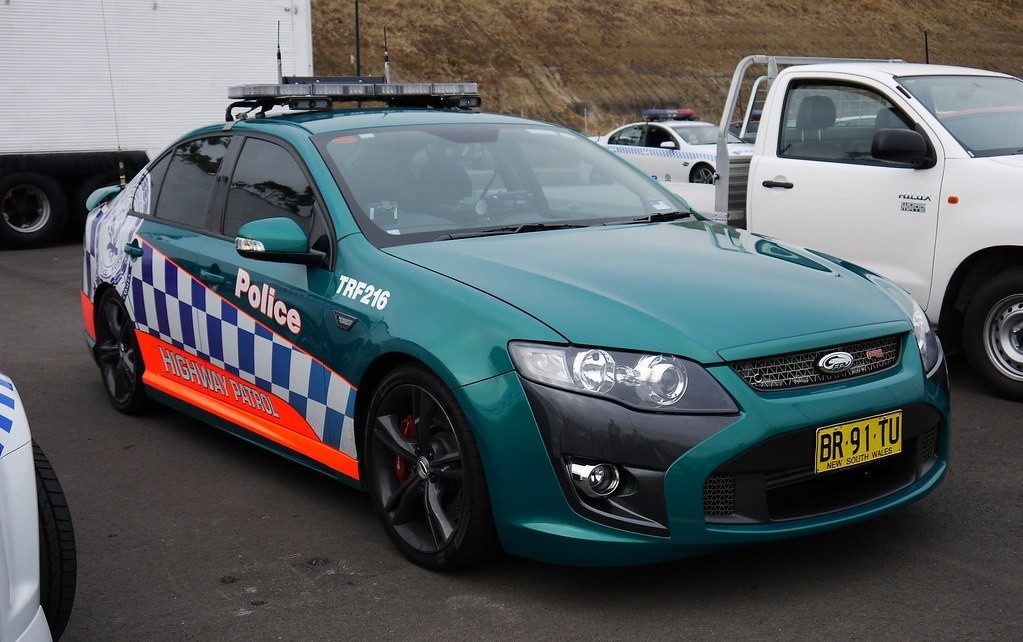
[786, 95, 840, 161]
[849, 106, 914, 154]
[413, 155, 472, 222]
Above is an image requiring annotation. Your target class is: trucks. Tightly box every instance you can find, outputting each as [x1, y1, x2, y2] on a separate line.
[0, 1, 317, 252]
[467, 56, 1023, 398]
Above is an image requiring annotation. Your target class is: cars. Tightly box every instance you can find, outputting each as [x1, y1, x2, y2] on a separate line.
[0, 371, 80, 642]
[833, 106, 1023, 126]
[79, 16, 952, 585]
[581, 108, 754, 183]
[726, 109, 764, 145]
